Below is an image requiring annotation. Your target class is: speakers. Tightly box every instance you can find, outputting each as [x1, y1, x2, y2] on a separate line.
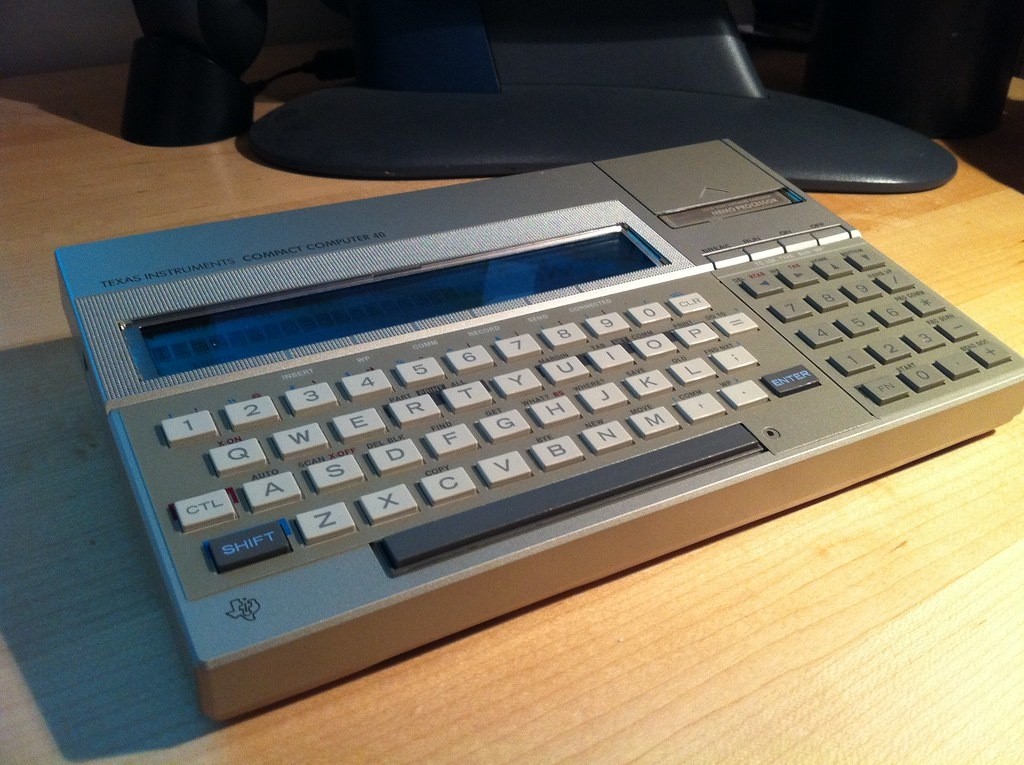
[802, 0, 1024, 141]
[121, 0, 269, 148]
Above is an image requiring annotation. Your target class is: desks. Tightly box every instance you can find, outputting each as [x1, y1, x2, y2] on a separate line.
[0, 62, 1024, 765]
